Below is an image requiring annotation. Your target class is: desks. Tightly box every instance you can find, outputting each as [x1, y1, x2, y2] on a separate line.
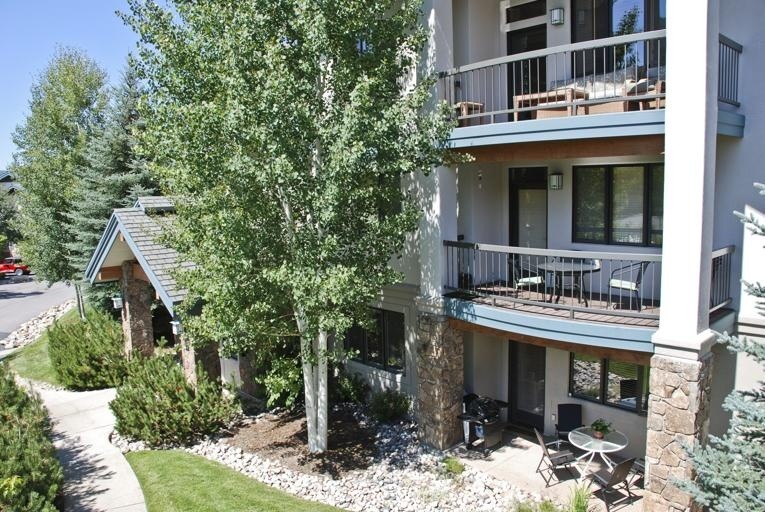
[536, 262, 600, 307]
[513, 88, 590, 121]
[569, 426, 630, 481]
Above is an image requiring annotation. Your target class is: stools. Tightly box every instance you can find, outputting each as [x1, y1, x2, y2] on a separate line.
[453, 101, 484, 125]
[624, 79, 665, 112]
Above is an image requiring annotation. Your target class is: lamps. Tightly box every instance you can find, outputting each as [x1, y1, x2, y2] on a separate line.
[111, 297, 125, 309]
[170, 321, 183, 335]
[550, 8, 564, 25]
[550, 173, 563, 191]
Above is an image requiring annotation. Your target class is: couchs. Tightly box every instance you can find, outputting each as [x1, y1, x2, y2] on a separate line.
[537, 65, 640, 118]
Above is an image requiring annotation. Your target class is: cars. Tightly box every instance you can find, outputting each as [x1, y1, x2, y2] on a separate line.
[0, 257, 31, 276]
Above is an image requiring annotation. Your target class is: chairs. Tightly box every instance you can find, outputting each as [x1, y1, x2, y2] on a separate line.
[507, 258, 546, 303]
[533, 427, 578, 488]
[629, 457, 645, 484]
[553, 404, 582, 451]
[605, 261, 651, 313]
[582, 457, 636, 512]
[549, 248, 585, 305]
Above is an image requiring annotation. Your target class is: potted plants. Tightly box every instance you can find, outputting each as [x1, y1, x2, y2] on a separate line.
[591, 418, 616, 439]
